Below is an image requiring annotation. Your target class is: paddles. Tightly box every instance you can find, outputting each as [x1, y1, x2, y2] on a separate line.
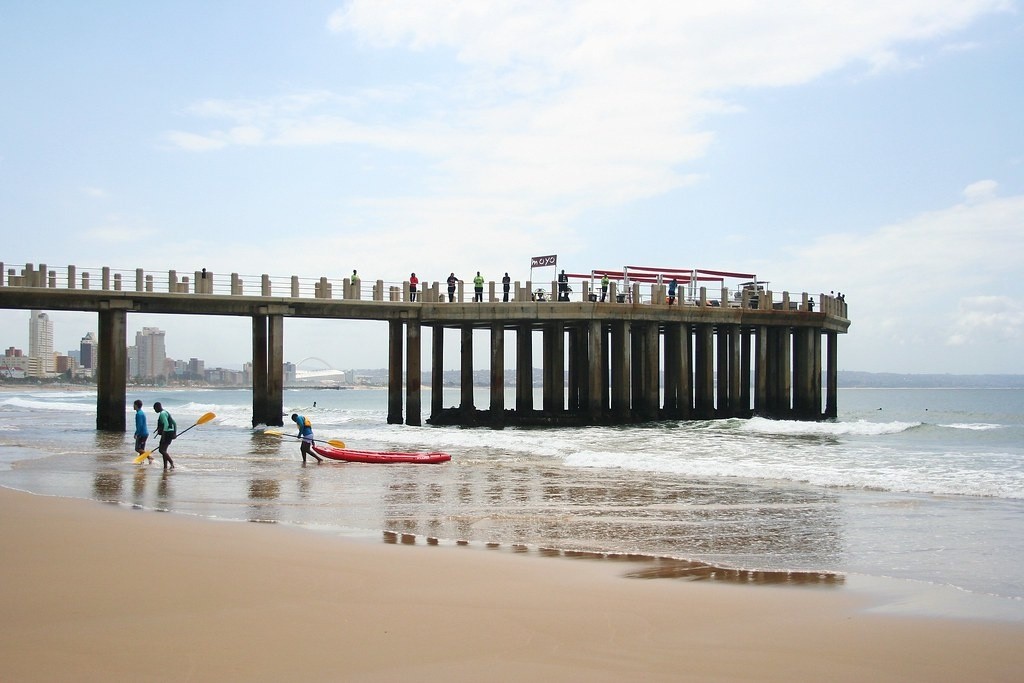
[264, 430, 345, 449]
[133, 412, 216, 463]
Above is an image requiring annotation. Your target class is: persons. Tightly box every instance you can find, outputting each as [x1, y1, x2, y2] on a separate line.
[313, 402, 316, 407]
[351, 270, 359, 285]
[600, 273, 609, 302]
[474, 271, 484, 302]
[502, 272, 510, 302]
[291, 414, 323, 465]
[410, 273, 418, 302]
[558, 270, 568, 301]
[447, 273, 458, 302]
[830, 291, 845, 302]
[202, 268, 206, 278]
[668, 279, 677, 305]
[153, 402, 177, 469]
[808, 297, 815, 311]
[134, 400, 154, 465]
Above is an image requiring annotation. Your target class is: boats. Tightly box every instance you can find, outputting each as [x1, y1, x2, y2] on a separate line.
[311, 446, 451, 463]
